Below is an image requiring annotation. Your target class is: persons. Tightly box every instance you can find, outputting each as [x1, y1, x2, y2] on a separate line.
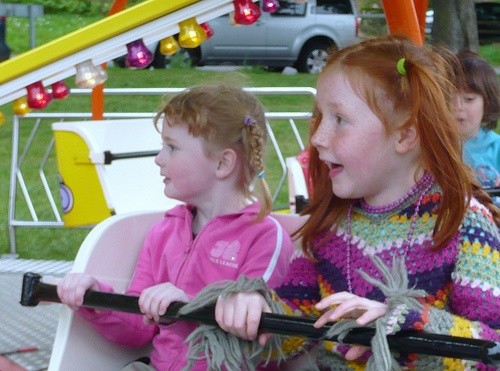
[444, 47, 500, 207]
[180, 36, 500, 371]
[56, 81, 296, 371]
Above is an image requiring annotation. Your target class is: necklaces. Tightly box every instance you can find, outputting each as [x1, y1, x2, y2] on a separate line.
[347, 171, 435, 304]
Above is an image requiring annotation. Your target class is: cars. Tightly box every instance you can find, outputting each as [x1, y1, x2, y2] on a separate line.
[113, 0, 361, 74]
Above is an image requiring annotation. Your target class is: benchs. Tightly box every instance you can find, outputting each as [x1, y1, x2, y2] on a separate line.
[8, 87, 318, 255]
[45, 211, 312, 371]
